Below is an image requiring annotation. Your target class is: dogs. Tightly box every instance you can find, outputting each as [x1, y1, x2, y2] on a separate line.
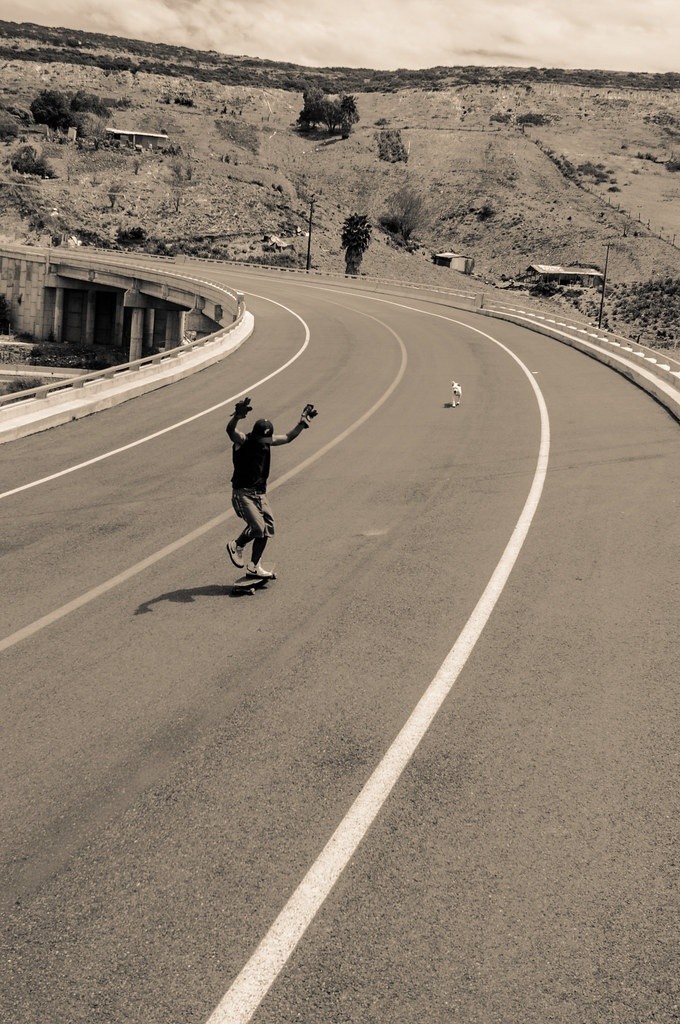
[451, 381, 461, 407]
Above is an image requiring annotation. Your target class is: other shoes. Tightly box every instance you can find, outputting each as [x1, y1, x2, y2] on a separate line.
[226, 539, 244, 569]
[246, 558, 272, 579]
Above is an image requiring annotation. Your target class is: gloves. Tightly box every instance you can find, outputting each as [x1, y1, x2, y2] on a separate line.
[234, 397, 252, 419]
[300, 404, 318, 429]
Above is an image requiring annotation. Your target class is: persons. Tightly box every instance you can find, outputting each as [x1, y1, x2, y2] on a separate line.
[226, 397, 318, 578]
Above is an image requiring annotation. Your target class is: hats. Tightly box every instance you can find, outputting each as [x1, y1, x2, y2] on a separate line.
[252, 418, 274, 445]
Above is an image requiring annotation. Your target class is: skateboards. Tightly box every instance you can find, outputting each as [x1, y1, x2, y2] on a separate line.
[231, 562, 278, 595]
